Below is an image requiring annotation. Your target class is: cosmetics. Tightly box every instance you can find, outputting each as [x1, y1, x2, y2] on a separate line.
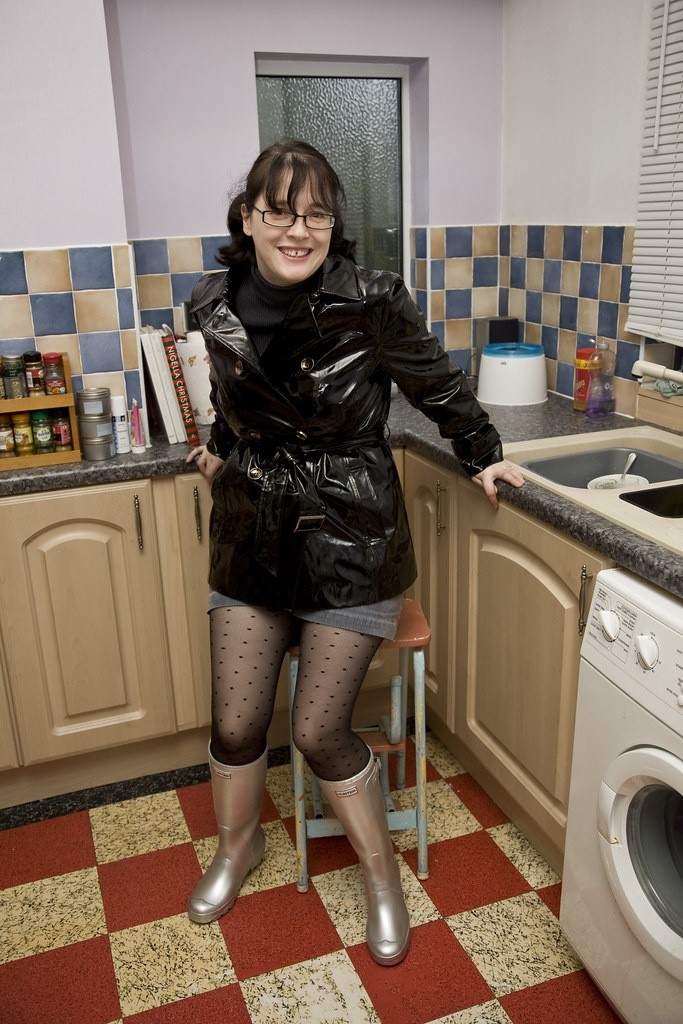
[112, 395, 132, 455]
[129, 399, 147, 455]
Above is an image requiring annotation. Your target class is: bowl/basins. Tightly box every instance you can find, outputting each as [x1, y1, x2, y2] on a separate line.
[587, 473, 649, 489]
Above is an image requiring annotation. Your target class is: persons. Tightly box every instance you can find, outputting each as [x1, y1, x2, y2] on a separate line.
[185, 141, 525, 966]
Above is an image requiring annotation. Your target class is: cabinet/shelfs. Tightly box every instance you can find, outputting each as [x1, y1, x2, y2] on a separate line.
[0, 448, 617, 884]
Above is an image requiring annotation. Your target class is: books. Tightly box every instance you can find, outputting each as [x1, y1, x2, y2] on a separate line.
[140, 323, 202, 447]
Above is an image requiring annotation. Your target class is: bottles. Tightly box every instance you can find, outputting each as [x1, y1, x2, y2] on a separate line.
[0, 350, 66, 398]
[110, 395, 131, 454]
[0, 409, 73, 458]
[585, 338, 617, 419]
[572, 347, 602, 412]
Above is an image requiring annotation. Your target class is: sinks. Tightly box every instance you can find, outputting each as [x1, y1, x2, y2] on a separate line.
[501, 424, 683, 496]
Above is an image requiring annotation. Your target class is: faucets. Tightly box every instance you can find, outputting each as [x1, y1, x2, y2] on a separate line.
[630, 359, 683, 385]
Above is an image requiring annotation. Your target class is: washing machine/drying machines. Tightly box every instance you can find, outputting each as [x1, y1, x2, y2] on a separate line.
[558, 564, 682, 1024]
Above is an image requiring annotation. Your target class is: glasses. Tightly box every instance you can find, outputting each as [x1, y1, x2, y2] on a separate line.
[245, 200, 336, 230]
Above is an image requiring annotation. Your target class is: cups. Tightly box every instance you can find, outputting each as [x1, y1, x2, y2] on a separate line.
[594, 477, 640, 488]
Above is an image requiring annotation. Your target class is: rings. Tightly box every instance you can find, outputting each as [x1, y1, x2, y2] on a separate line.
[502, 469, 507, 476]
[200, 455, 205, 459]
[507, 465, 514, 471]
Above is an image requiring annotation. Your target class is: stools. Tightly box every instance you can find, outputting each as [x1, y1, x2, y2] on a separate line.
[288, 598, 431, 894]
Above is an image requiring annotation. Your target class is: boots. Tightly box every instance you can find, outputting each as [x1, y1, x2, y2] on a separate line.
[188, 739, 268, 923]
[314, 743, 410, 966]
